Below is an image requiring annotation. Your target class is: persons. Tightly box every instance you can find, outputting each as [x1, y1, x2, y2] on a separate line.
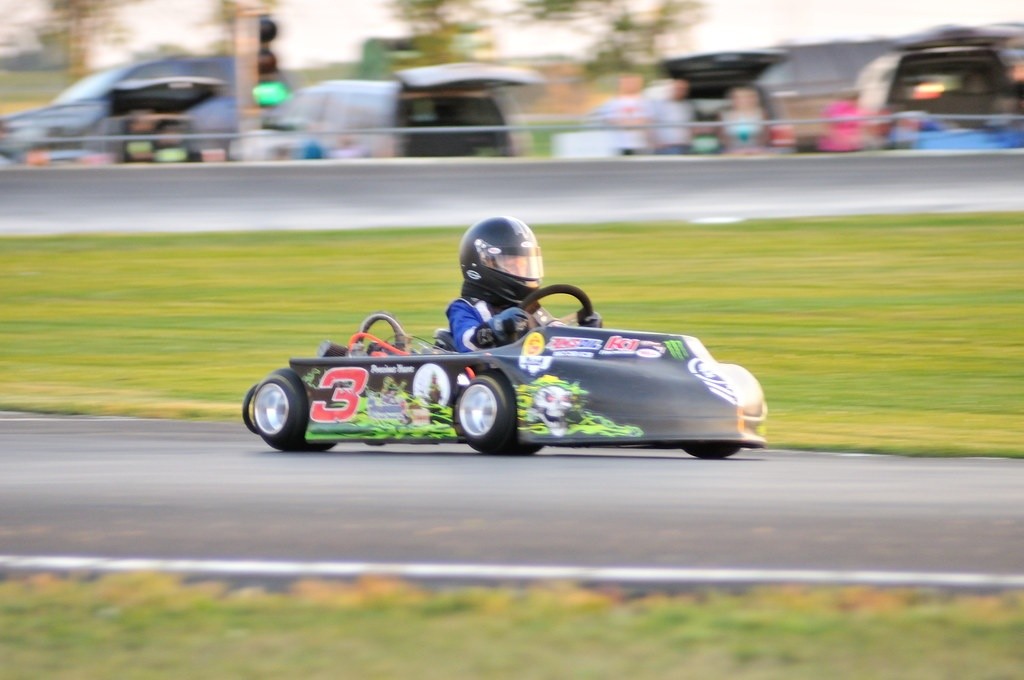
[446, 217, 604, 353]
[122, 110, 203, 164]
[592, 72, 770, 153]
[817, 89, 865, 152]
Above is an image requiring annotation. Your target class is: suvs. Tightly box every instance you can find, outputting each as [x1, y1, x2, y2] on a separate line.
[0, 51, 280, 167]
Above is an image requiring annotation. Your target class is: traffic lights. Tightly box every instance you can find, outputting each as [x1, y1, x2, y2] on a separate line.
[236, 14, 288, 111]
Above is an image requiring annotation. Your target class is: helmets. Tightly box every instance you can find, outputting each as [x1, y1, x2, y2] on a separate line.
[458, 216, 543, 310]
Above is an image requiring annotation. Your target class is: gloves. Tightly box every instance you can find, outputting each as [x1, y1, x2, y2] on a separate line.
[585, 313, 603, 328]
[488, 307, 530, 337]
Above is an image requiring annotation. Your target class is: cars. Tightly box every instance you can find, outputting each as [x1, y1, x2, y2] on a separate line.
[850, 22, 1024, 152]
[557, 46, 797, 156]
[228, 61, 547, 164]
